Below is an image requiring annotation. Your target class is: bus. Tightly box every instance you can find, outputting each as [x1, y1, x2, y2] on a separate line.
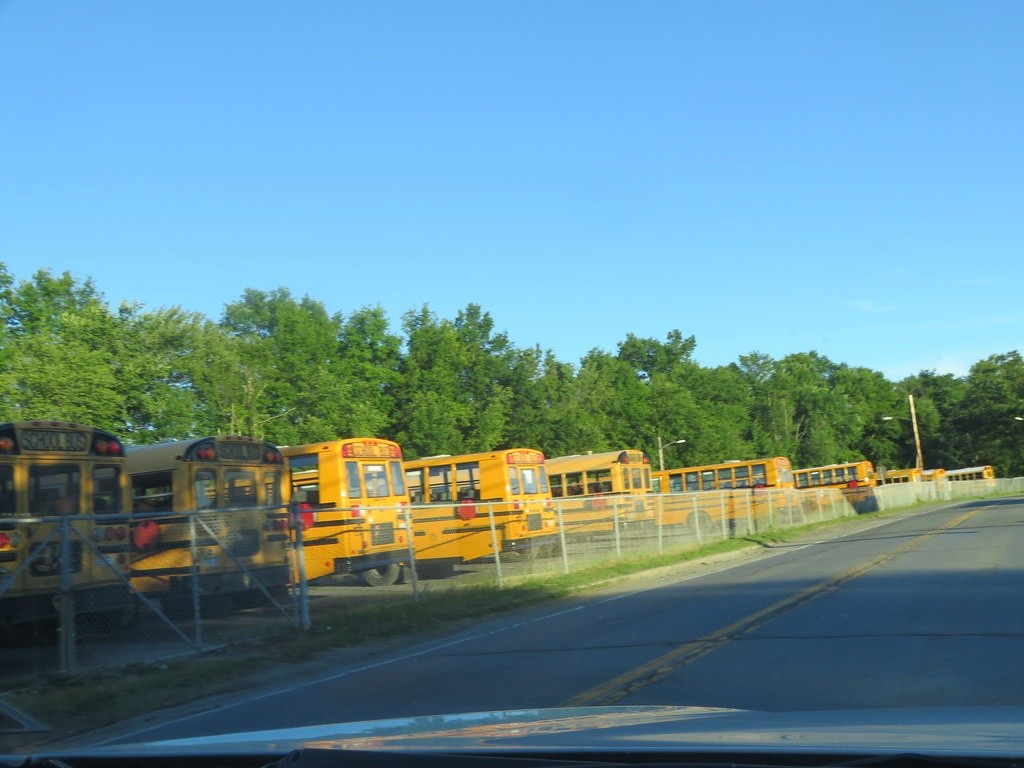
[815, 468, 921, 487]
[921, 469, 952, 493]
[702, 461, 875, 513]
[5, 435, 292, 619]
[945, 466, 996, 490]
[191, 437, 413, 587]
[0, 421, 130, 638]
[408, 450, 655, 540]
[572, 458, 799, 537]
[204, 448, 555, 567]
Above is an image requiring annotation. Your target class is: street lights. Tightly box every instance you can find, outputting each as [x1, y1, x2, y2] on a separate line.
[658, 436, 686, 470]
[882, 394, 923, 469]
